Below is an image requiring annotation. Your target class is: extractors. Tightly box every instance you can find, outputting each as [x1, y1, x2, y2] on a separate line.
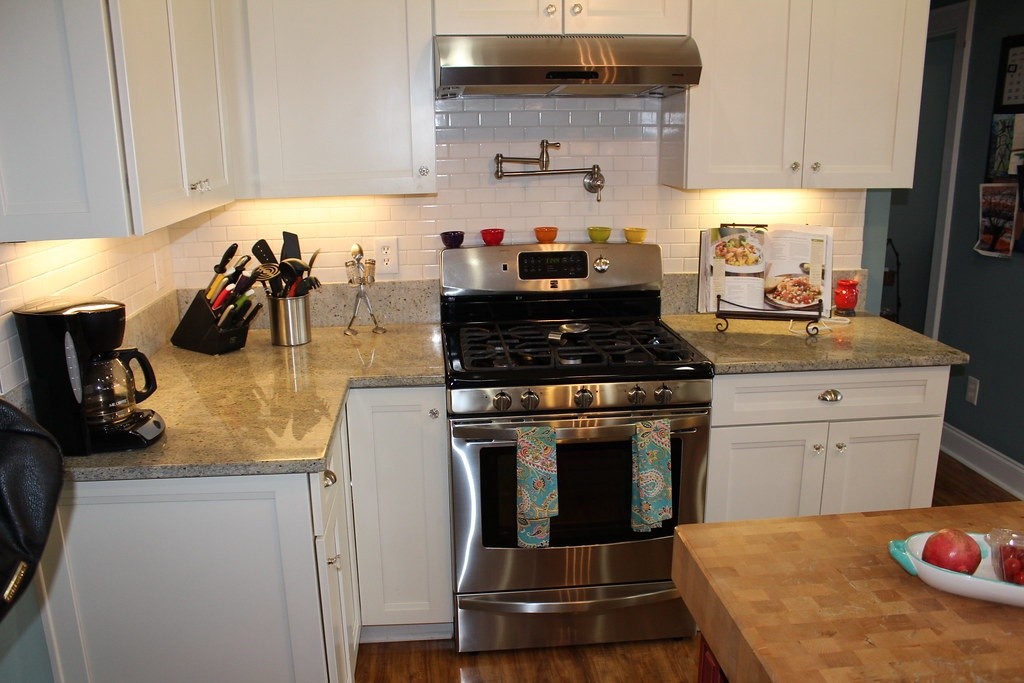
[432, 34, 702, 99]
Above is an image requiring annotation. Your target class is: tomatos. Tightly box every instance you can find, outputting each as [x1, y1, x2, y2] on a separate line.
[998, 543, 1024, 586]
[922, 528, 982, 577]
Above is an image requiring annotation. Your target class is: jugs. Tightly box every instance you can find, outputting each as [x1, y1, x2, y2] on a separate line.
[81, 348, 156, 421]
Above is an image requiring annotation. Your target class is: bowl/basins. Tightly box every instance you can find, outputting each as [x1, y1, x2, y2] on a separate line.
[480, 228, 505, 246]
[587, 227, 611, 243]
[440, 231, 465, 248]
[534, 226, 558, 243]
[985, 527, 1024, 584]
[624, 227, 647, 244]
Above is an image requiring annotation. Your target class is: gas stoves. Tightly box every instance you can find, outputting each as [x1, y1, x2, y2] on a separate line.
[439, 240, 715, 409]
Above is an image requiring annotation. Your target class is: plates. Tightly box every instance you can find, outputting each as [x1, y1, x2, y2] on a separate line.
[766, 285, 824, 310]
[887, 530, 1024, 607]
[709, 232, 766, 273]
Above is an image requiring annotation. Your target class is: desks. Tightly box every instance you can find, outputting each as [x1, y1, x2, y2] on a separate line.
[670, 500, 1024, 683]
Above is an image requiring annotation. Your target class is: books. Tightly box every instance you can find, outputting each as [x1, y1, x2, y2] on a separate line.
[701, 227, 827, 316]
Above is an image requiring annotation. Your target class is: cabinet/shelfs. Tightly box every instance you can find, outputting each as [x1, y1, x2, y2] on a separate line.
[704, 365, 951, 521]
[0, 0, 437, 241]
[658, 0, 931, 190]
[880, 238, 900, 324]
[433, 0, 690, 37]
[31, 383, 455, 683]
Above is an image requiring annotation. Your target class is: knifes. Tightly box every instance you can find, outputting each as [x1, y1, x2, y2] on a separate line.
[203, 243, 263, 331]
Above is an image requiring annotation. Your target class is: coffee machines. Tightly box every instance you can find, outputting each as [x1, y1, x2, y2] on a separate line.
[12, 293, 166, 456]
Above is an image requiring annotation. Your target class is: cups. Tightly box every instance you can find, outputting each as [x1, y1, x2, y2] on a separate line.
[266, 294, 311, 346]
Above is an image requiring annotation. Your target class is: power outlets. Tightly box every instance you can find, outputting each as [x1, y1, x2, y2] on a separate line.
[965, 376, 980, 405]
[373, 237, 400, 274]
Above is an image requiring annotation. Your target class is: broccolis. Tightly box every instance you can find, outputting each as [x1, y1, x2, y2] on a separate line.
[723, 235, 749, 247]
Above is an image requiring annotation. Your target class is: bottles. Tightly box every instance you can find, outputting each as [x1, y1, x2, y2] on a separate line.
[834, 280, 859, 315]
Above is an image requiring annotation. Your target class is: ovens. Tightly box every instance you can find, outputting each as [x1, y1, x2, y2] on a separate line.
[449, 412, 711, 652]
